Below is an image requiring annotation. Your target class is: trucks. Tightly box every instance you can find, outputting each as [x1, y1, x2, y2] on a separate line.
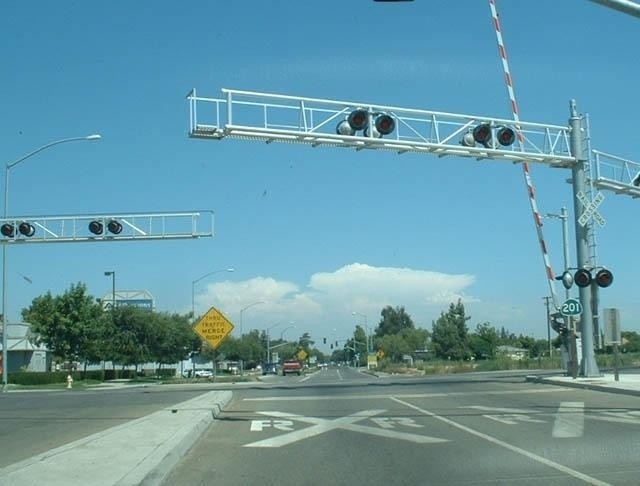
[218, 360, 238, 375]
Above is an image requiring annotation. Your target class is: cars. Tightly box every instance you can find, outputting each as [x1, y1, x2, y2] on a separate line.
[186, 367, 214, 381]
[262, 363, 277, 376]
[174, 367, 194, 379]
[282, 360, 302, 377]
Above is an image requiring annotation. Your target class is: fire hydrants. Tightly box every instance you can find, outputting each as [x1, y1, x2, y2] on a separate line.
[65, 374, 74, 390]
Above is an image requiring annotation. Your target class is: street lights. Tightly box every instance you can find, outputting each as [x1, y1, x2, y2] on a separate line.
[105, 269, 116, 378]
[266, 310, 374, 370]
[190, 267, 234, 375]
[238, 301, 269, 377]
[3, 133, 102, 385]
[543, 205, 574, 330]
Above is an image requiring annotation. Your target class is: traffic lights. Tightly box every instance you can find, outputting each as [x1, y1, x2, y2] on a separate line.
[346, 108, 369, 129]
[473, 124, 492, 145]
[496, 126, 516, 146]
[18, 222, 29, 235]
[376, 115, 394, 134]
[594, 269, 613, 288]
[574, 266, 591, 289]
[0, 223, 11, 238]
[88, 220, 101, 235]
[108, 220, 119, 233]
